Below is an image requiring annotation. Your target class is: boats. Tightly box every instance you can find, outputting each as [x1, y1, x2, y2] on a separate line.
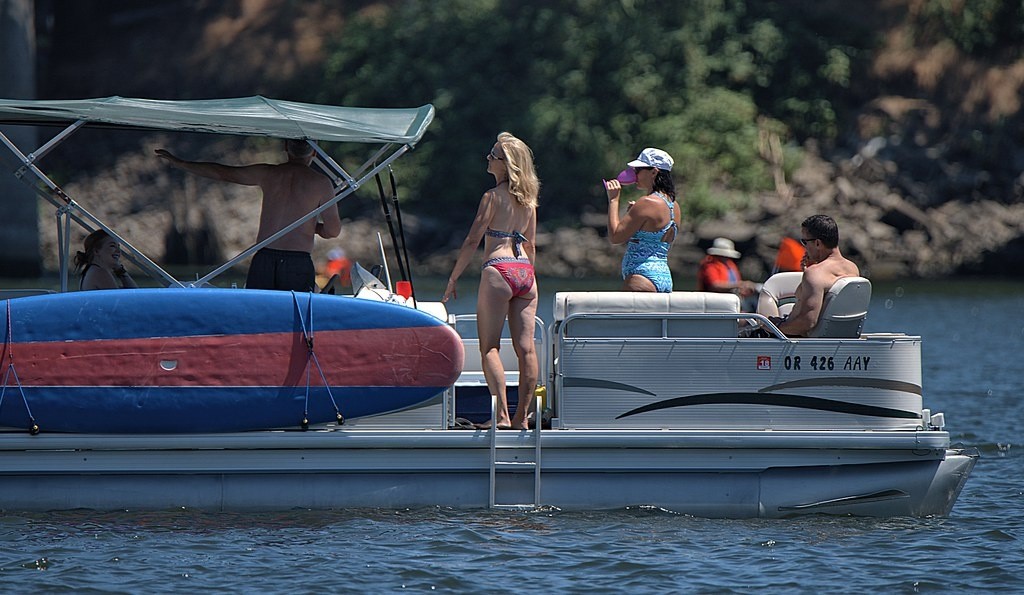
[0, 96, 978, 515]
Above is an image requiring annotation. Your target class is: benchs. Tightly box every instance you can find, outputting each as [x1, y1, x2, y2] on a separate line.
[552, 289, 740, 322]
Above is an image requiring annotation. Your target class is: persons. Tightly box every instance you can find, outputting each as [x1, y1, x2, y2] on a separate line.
[738, 214, 859, 338]
[154, 139, 341, 292]
[697, 238, 755, 307]
[74, 229, 137, 290]
[442, 133, 538, 429]
[607, 148, 680, 292]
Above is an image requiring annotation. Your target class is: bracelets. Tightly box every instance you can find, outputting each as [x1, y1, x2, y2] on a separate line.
[114, 265, 127, 277]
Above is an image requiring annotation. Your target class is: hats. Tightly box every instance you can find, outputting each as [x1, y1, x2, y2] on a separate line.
[707, 237, 741, 258]
[627, 148, 675, 171]
[776, 238, 805, 271]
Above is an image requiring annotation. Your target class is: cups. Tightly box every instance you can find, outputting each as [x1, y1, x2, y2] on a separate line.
[395, 280, 413, 308]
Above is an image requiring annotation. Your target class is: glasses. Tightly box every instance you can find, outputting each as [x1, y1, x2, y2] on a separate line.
[490, 151, 505, 160]
[634, 167, 654, 174]
[801, 239, 817, 246]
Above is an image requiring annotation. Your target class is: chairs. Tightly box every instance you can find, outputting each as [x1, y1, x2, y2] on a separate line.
[806, 275, 871, 338]
[757, 272, 803, 325]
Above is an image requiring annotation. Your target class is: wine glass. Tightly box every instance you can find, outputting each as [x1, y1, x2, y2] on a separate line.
[602, 167, 637, 190]
